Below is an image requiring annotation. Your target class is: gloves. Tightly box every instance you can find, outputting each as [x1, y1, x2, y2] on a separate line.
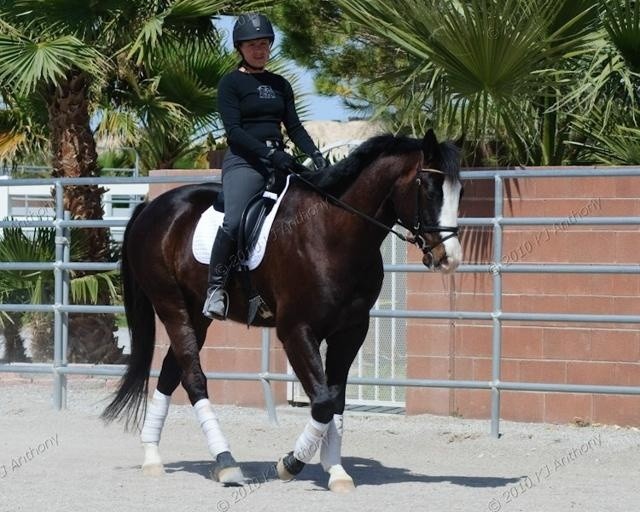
[312, 150, 328, 171]
[270, 150, 296, 172]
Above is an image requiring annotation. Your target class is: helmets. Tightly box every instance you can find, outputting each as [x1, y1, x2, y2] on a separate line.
[232, 11, 275, 48]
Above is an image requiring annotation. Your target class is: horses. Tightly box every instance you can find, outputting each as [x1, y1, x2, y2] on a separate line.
[98, 127, 467, 493]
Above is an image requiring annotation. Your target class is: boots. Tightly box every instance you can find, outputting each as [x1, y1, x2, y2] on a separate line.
[202, 227, 236, 319]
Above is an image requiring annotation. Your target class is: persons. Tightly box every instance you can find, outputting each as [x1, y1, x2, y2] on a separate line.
[202, 13, 329, 320]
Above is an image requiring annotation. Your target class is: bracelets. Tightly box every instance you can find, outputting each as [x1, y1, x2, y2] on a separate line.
[266, 148, 277, 158]
[314, 150, 320, 153]
